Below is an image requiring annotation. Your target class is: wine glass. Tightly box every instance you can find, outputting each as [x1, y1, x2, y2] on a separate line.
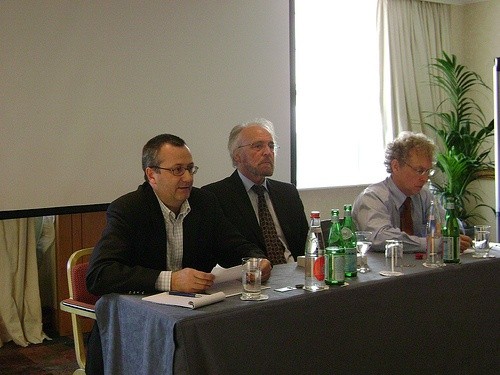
[355, 231, 373, 272]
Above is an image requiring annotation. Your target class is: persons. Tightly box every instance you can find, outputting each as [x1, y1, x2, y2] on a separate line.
[84, 133, 273, 375]
[352, 130, 475, 257]
[200, 116, 320, 265]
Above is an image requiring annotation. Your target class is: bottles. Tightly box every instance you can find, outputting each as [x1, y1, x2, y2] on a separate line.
[324, 208, 348, 283]
[442, 197, 461, 263]
[305, 211, 328, 289]
[341, 204, 359, 276]
[427, 200, 445, 266]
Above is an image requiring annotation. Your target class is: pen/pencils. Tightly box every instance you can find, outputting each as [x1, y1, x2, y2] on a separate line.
[456, 218, 474, 249]
[169, 292, 202, 298]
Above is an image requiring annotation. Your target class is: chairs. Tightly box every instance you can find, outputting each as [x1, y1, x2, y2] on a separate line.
[59, 247, 96, 375]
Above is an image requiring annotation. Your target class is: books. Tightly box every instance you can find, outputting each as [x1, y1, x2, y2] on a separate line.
[142, 291, 226, 309]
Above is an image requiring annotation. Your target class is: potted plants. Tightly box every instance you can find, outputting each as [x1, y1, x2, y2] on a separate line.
[411, 50, 495, 237]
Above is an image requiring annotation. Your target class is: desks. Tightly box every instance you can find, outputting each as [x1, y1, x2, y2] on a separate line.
[95, 252, 500, 375]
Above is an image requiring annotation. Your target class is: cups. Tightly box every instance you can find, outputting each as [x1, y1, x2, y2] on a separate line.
[241, 257, 261, 299]
[473, 225, 493, 257]
[385, 240, 403, 260]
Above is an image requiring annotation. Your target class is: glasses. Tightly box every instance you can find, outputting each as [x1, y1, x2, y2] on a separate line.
[237, 141, 280, 153]
[143, 165, 199, 176]
[393, 157, 433, 176]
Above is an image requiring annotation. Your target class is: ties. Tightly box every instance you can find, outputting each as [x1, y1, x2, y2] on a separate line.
[399, 197, 413, 236]
[250, 184, 287, 266]
